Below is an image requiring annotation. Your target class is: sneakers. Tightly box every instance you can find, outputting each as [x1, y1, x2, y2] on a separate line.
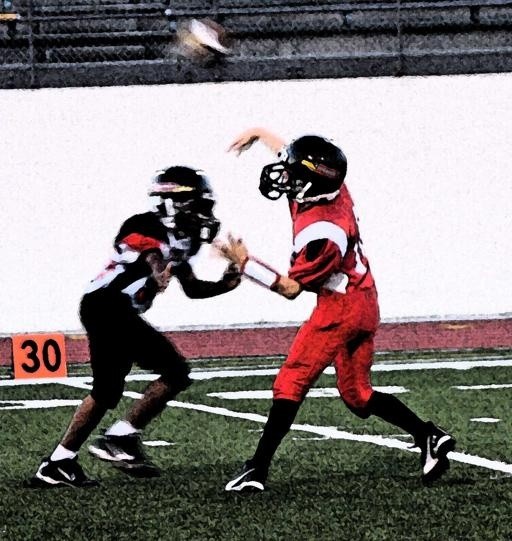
[85, 428, 162, 482]
[34, 453, 100, 491]
[223, 459, 267, 494]
[413, 420, 457, 481]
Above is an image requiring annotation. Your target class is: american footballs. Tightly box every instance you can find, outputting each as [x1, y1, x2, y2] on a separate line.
[163, 18, 233, 70]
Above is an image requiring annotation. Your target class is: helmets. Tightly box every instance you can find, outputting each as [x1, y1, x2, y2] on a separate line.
[279, 133, 348, 207]
[146, 165, 212, 233]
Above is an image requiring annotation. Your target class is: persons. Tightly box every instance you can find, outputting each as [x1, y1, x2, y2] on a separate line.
[37, 166, 243, 491]
[207, 127, 456, 496]
